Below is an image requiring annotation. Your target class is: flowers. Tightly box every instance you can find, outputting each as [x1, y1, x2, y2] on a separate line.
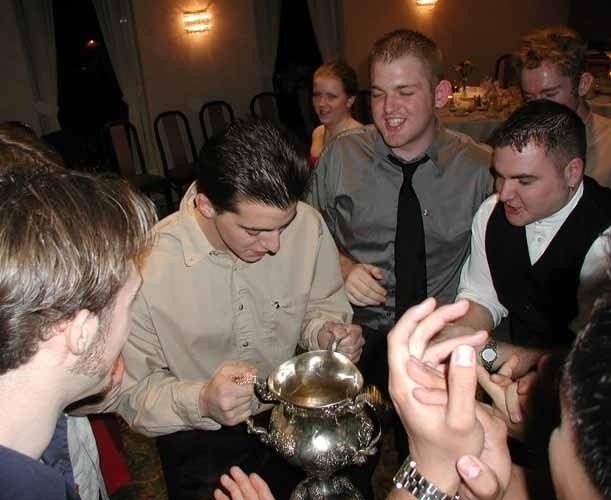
[453, 57, 475, 89]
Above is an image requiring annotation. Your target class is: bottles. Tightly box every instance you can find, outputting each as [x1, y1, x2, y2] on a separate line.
[439, 74, 523, 121]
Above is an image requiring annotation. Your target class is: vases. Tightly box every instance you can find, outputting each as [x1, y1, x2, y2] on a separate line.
[458, 79, 470, 98]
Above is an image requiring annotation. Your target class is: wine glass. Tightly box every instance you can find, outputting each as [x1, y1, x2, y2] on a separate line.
[236, 332, 383, 500]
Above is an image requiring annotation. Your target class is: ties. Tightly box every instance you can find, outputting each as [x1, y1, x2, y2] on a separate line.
[387, 153, 430, 325]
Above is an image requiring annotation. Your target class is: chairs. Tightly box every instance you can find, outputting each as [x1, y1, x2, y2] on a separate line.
[491, 52, 525, 89]
[102, 91, 285, 214]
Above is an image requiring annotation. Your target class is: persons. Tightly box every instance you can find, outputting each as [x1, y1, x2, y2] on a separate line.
[308, 26, 610, 500]
[2, 118, 362, 499]
